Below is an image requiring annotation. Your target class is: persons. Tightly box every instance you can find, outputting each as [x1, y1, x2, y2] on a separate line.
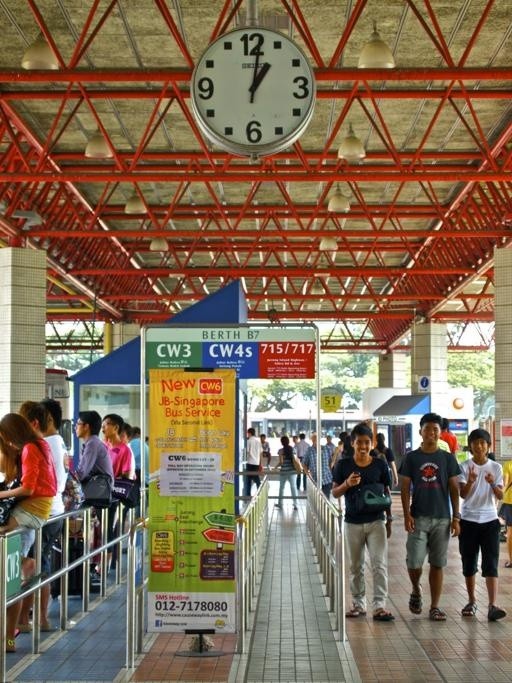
[399, 412, 462, 621]
[331, 423, 396, 621]
[499, 461, 512, 568]
[458, 428, 507, 621]
[245, 417, 456, 509]
[0, 398, 149, 654]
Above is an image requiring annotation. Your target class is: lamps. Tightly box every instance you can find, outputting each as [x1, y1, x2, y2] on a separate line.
[21, 29, 170, 251]
[316, 19, 396, 254]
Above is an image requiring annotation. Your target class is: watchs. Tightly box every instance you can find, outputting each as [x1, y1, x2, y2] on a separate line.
[386, 515, 393, 521]
[453, 512, 461, 521]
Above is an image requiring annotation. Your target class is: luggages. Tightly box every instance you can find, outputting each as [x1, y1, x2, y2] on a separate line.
[50, 536, 82, 599]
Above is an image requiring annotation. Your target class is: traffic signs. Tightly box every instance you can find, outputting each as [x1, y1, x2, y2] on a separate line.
[201, 526, 237, 545]
[201, 510, 236, 529]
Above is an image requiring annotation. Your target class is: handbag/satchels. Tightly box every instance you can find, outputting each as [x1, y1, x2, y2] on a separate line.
[362, 491, 392, 513]
[0, 482, 25, 527]
[61, 472, 140, 510]
[69, 518, 84, 537]
[291, 455, 303, 474]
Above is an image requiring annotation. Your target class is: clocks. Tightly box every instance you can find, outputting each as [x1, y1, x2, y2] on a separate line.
[183, 23, 321, 161]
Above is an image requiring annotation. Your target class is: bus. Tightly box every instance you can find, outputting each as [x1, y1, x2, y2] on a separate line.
[247, 406, 391, 457]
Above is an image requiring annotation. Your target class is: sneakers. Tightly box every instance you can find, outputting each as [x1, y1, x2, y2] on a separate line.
[6, 640, 16, 652]
[15, 623, 32, 633]
[40, 623, 51, 632]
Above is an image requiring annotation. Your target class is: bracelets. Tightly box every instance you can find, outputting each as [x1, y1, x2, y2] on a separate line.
[491, 483, 495, 488]
[345, 479, 349, 488]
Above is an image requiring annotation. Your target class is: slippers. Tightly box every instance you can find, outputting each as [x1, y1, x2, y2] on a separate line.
[462, 603, 477, 616]
[488, 605, 506, 620]
[409, 584, 423, 614]
[430, 607, 446, 621]
[345, 606, 367, 618]
[374, 610, 395, 621]
